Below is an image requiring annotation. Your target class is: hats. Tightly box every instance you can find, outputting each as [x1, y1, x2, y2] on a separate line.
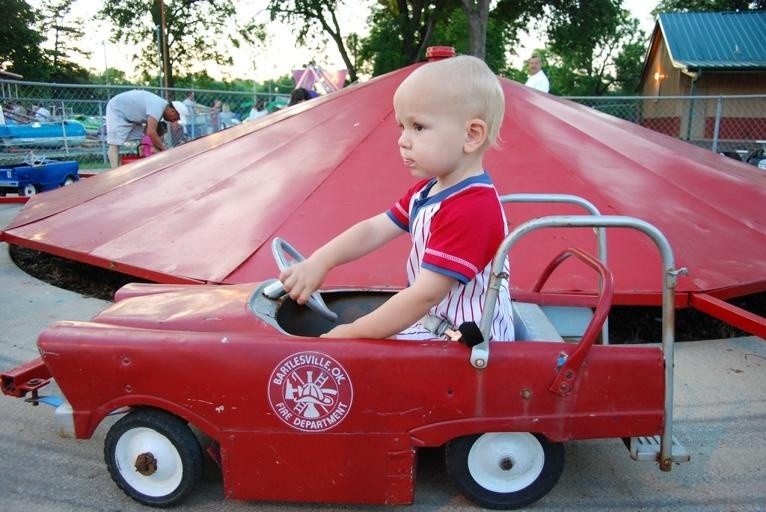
[172, 101, 189, 125]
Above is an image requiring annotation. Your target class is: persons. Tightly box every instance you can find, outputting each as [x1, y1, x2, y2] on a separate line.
[217, 102, 235, 129]
[103, 88, 188, 168]
[524, 54, 550, 92]
[287, 87, 309, 110]
[0, 99, 50, 125]
[249, 98, 268, 120]
[208, 100, 220, 133]
[138, 121, 167, 156]
[182, 91, 198, 140]
[277, 54, 515, 344]
[169, 121, 188, 147]
